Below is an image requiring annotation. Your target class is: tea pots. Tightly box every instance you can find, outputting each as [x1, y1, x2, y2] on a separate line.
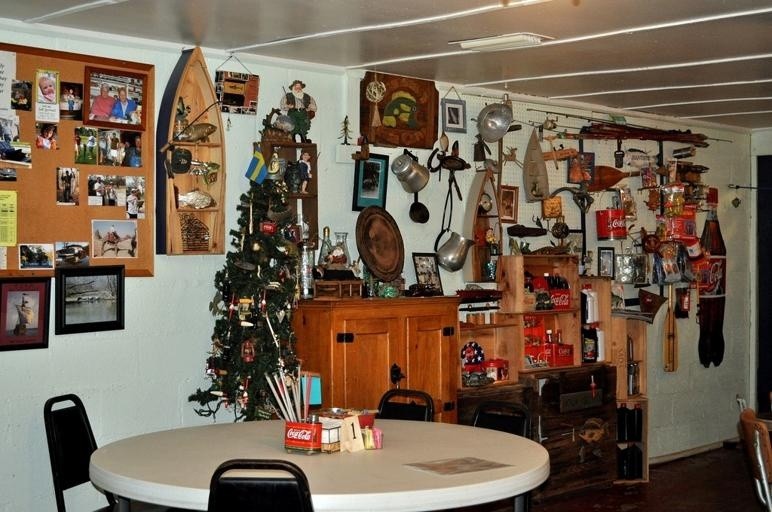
[432, 229, 477, 272]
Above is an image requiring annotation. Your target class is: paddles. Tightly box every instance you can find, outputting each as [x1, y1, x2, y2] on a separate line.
[662, 284, 679, 373]
[587, 165, 666, 192]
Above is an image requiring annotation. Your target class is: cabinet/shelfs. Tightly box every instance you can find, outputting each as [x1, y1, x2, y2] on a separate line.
[290, 295, 462, 424]
[172, 141, 222, 212]
[460, 254, 649, 504]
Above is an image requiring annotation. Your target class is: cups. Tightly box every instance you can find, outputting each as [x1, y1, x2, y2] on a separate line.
[466, 311, 505, 325]
[394, 149, 429, 195]
[295, 240, 316, 299]
[174, 120, 189, 142]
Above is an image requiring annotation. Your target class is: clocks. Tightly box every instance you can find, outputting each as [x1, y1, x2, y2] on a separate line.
[479, 193, 493, 213]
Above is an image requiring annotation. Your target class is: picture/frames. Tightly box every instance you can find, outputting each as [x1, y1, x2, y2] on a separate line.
[497, 185, 519, 223]
[352, 150, 389, 212]
[55, 264, 125, 335]
[83, 65, 148, 131]
[0, 276, 51, 352]
[441, 86, 467, 134]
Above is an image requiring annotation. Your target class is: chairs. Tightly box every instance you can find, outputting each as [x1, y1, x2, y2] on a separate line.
[44, 394, 119, 512]
[375, 388, 435, 422]
[740, 408, 772, 512]
[207, 459, 314, 512]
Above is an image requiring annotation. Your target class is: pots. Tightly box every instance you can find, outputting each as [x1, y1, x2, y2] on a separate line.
[410, 191, 429, 225]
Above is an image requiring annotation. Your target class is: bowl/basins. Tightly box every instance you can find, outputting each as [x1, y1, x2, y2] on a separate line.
[477, 103, 513, 143]
[319, 407, 375, 428]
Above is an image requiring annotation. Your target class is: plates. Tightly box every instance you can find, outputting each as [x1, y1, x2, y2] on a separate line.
[356, 206, 404, 281]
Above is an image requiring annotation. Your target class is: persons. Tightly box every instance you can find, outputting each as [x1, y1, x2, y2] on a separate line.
[279, 80, 318, 144]
[0, 76, 141, 267]
[297, 151, 313, 194]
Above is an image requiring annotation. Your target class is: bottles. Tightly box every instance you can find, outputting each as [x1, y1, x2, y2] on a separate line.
[362, 426, 383, 449]
[581, 281, 605, 364]
[525, 271, 570, 310]
[318, 226, 350, 271]
[482, 358, 509, 381]
[617, 403, 644, 479]
[308, 413, 319, 424]
[696, 187, 728, 368]
[626, 334, 641, 396]
[545, 330, 557, 343]
[555, 328, 563, 344]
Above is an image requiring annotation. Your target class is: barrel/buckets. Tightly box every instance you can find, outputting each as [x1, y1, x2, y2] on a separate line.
[594, 189, 627, 240]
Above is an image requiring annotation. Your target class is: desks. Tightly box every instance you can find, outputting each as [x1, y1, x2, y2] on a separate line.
[89, 419, 550, 512]
